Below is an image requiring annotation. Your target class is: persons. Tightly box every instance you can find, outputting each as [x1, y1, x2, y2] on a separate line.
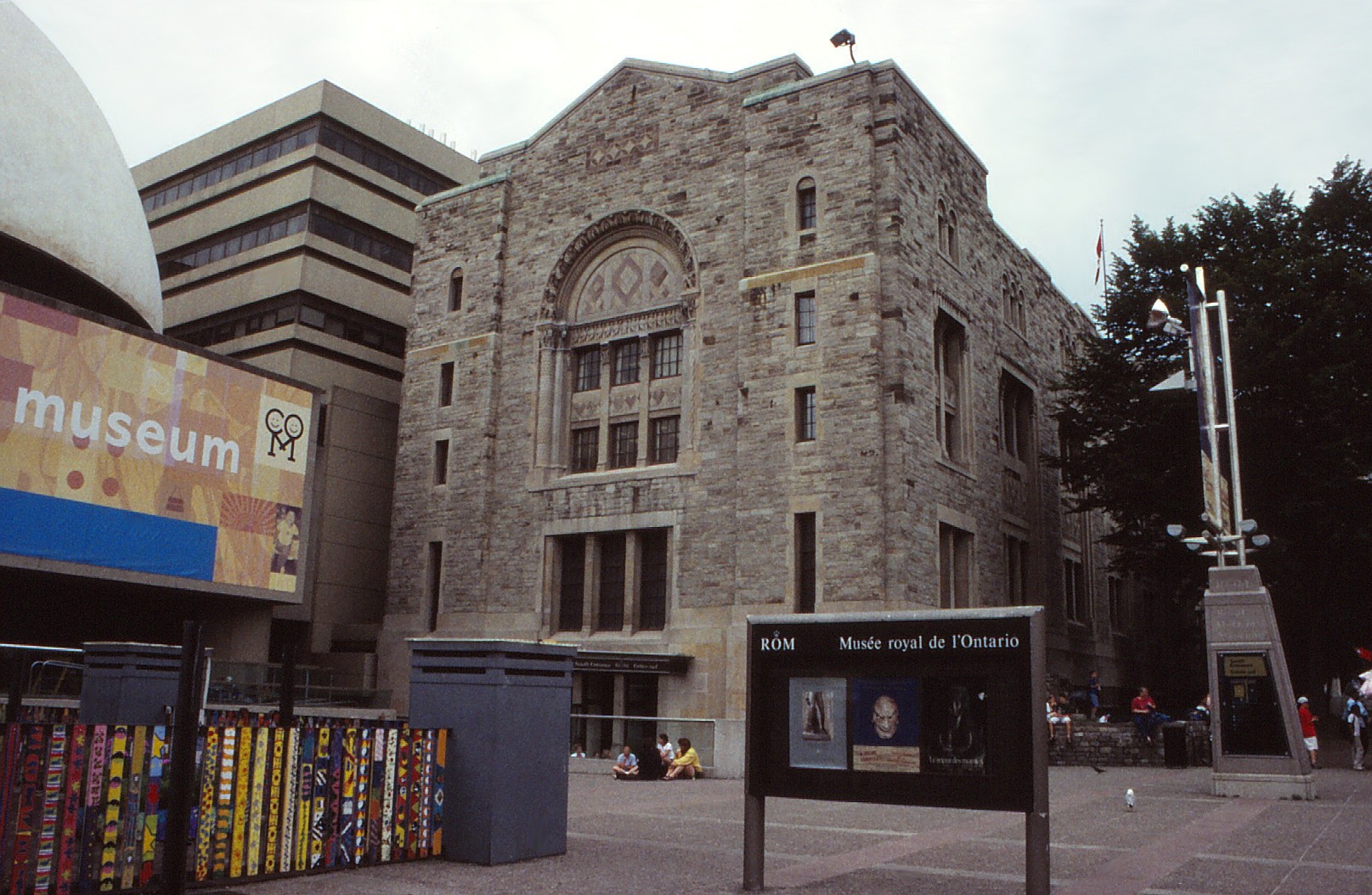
[1296, 696, 1322, 768]
[1131, 686, 1156, 741]
[570, 745, 586, 758]
[612, 745, 638, 775]
[662, 738, 703, 780]
[1086, 670, 1100, 721]
[1046, 688, 1073, 744]
[656, 733, 675, 767]
[1342, 686, 1367, 770]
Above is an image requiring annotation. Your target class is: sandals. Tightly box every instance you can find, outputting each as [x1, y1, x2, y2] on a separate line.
[1066, 736, 1072, 744]
[1047, 735, 1056, 742]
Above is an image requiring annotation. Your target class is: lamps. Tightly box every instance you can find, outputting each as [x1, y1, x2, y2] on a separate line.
[830, 28, 856, 64]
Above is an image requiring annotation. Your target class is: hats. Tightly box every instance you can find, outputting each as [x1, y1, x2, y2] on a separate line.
[1297, 696, 1308, 704]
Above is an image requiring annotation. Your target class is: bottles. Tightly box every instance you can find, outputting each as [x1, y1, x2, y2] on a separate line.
[1125, 788, 1136, 812]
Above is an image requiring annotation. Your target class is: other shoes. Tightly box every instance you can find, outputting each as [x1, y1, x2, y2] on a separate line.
[1311, 762, 1322, 769]
[1353, 763, 1366, 770]
